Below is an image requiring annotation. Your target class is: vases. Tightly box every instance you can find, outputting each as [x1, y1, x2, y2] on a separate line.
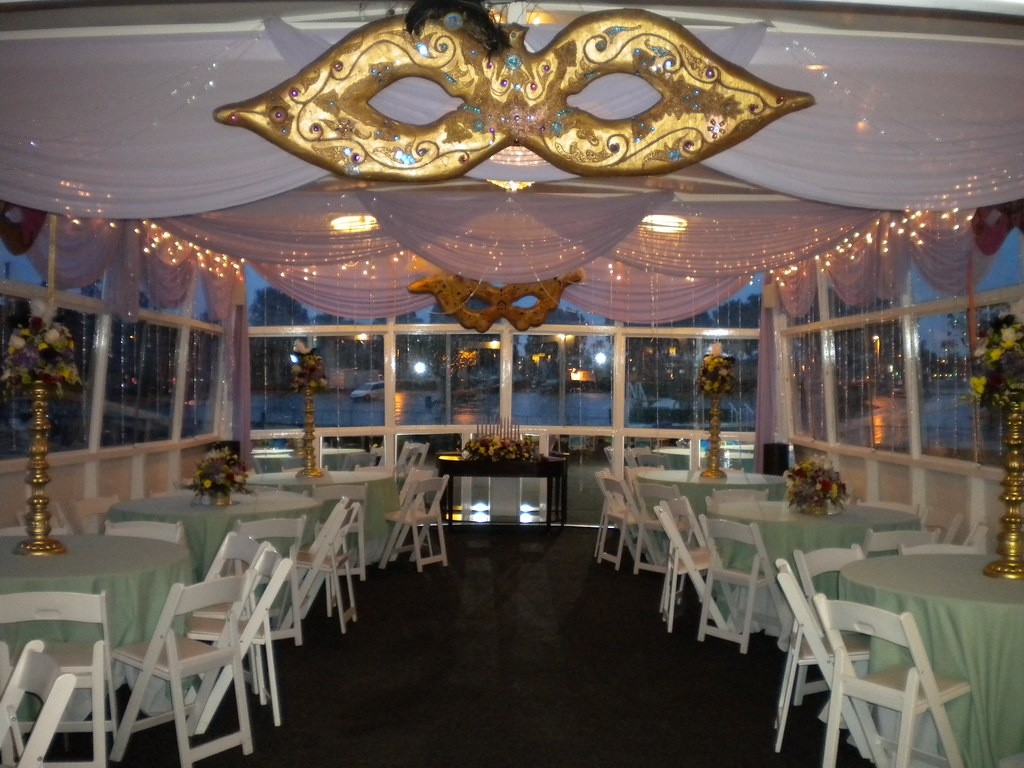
[805, 502, 828, 516]
[210, 491, 233, 506]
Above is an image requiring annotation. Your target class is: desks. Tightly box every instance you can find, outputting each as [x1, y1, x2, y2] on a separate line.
[107, 490, 323, 617]
[249, 449, 295, 455]
[253, 449, 365, 472]
[637, 470, 788, 567]
[706, 502, 921, 622]
[439, 455, 567, 538]
[839, 555, 1024, 768]
[0, 535, 203, 738]
[435, 452, 575, 520]
[651, 448, 754, 474]
[720, 445, 754, 453]
[245, 472, 402, 565]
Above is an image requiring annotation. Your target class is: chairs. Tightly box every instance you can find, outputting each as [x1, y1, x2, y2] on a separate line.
[0, 464, 449, 768]
[370, 441, 430, 468]
[604, 444, 651, 464]
[595, 466, 988, 767]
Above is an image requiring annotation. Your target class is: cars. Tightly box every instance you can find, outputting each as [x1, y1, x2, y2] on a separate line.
[349, 380, 385, 402]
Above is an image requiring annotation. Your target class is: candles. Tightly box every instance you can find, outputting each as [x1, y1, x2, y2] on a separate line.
[470, 415, 522, 441]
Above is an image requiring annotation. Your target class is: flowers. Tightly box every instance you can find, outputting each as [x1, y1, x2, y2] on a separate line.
[456, 436, 549, 464]
[783, 453, 851, 513]
[694, 344, 736, 396]
[183, 447, 258, 508]
[285, 340, 328, 396]
[0, 317, 83, 400]
[960, 309, 1024, 409]
